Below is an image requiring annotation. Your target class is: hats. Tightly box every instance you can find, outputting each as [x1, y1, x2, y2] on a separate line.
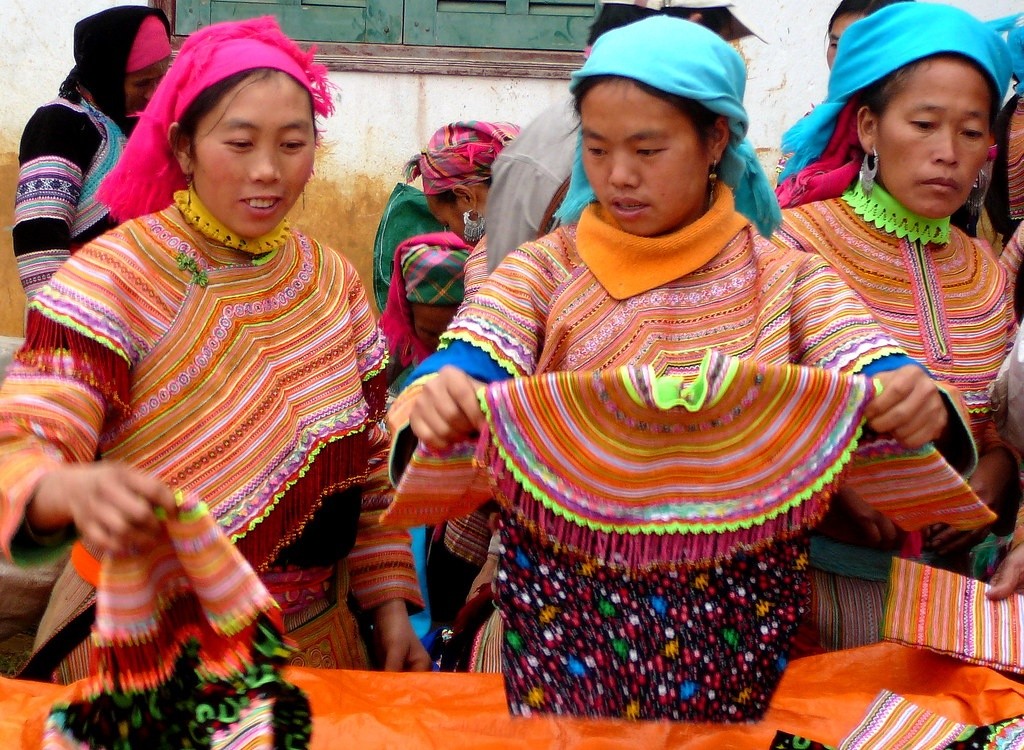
[125, 13, 172, 72]
[401, 242, 469, 304]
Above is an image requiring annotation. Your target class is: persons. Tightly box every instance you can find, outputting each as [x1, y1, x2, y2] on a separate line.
[0, 0, 1024, 669]
